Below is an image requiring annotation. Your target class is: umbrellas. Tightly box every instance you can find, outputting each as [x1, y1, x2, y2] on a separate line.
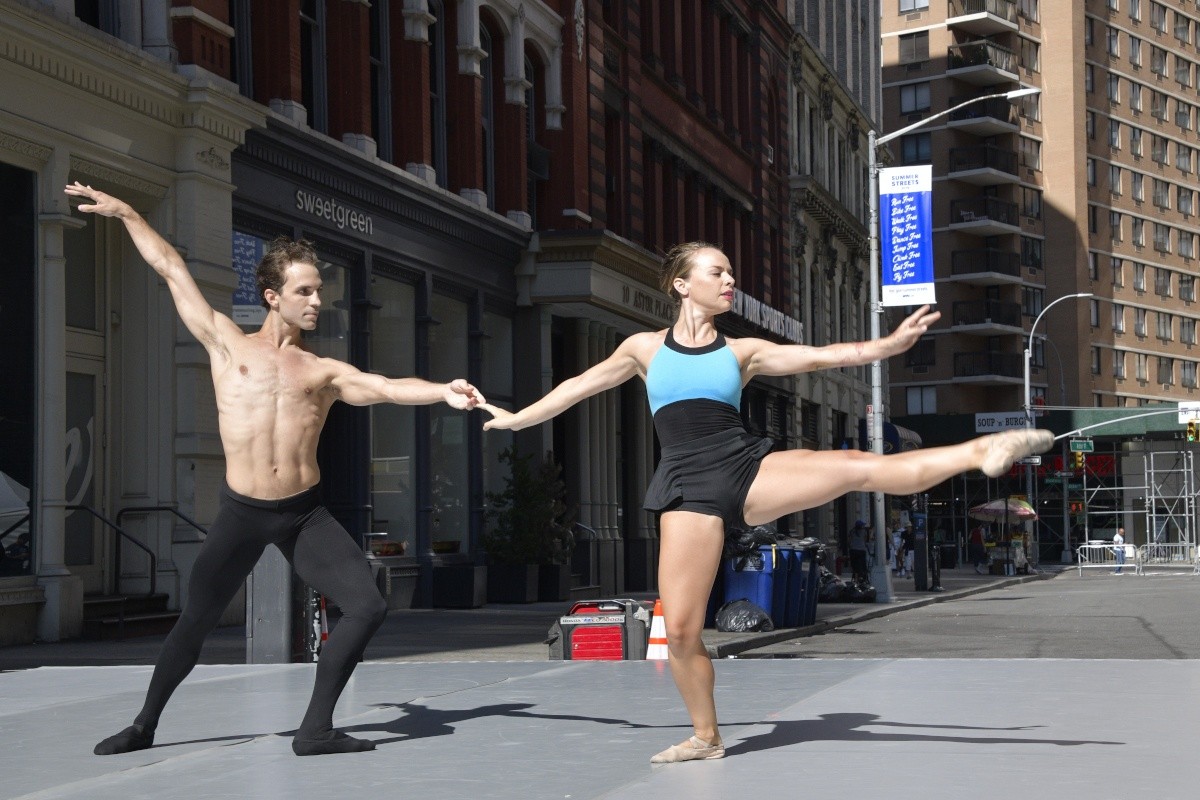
[969, 498, 1036, 542]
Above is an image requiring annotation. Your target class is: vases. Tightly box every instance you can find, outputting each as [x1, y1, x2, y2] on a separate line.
[433, 564, 488, 609]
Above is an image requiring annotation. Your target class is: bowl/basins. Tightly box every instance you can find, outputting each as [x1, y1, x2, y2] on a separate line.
[370, 542, 405, 556]
[432, 541, 462, 554]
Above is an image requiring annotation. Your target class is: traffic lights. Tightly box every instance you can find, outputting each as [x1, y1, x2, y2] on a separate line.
[1075, 452, 1084, 470]
[1186, 420, 1197, 442]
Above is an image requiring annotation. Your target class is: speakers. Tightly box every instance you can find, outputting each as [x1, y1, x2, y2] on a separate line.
[548, 600, 655, 659]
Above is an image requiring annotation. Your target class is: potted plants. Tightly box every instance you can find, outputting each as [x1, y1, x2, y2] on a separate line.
[472, 442, 580, 603]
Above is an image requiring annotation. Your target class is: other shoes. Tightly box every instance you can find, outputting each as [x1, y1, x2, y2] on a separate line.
[896, 568, 905, 577]
[1114, 572, 1124, 575]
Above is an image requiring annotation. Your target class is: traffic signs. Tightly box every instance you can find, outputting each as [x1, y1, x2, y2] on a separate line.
[1016, 455, 1041, 466]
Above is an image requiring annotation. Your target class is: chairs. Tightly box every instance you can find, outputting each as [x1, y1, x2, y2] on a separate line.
[960, 210, 988, 223]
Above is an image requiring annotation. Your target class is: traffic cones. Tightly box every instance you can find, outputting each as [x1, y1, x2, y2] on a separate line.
[646, 599, 670, 661]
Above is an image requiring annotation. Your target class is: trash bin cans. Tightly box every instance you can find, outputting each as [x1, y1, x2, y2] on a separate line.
[545, 596, 653, 659]
[705, 537, 825, 628]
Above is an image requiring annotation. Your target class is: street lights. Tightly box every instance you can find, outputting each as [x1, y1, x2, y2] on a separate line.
[865, 86, 1045, 607]
[1023, 291, 1095, 568]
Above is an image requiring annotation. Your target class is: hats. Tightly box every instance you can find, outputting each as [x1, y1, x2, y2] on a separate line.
[855, 520, 866, 527]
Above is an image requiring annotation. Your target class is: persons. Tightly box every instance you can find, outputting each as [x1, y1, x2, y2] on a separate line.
[472, 239, 1056, 768]
[848, 519, 915, 584]
[65, 181, 487, 757]
[968, 522, 986, 574]
[1112, 529, 1126, 576]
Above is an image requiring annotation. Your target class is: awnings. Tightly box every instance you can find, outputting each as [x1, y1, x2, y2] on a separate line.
[883, 421, 922, 448]
